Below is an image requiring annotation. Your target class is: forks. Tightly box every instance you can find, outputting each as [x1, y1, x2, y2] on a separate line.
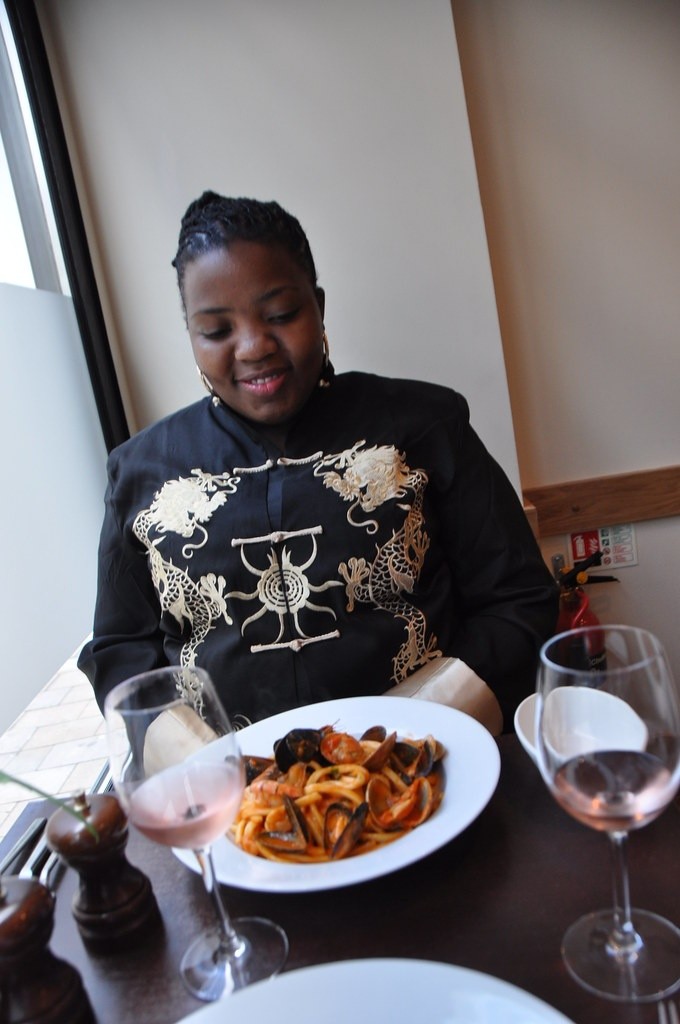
[657, 1001, 680, 1024]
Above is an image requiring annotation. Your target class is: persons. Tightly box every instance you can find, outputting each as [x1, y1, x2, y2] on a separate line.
[76, 190, 563, 781]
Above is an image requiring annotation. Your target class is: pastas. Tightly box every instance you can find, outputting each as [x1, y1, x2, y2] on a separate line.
[232, 763, 411, 864]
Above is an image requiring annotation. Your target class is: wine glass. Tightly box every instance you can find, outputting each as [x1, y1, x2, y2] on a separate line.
[536, 623, 680, 1002]
[104, 661, 288, 1003]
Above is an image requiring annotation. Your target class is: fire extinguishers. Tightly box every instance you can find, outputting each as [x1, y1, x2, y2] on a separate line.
[554, 550, 621, 690]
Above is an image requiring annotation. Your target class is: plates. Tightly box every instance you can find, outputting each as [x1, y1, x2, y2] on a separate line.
[169, 695, 502, 892]
[172, 956, 574, 1024]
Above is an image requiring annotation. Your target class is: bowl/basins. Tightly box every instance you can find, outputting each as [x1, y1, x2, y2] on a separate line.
[514, 684, 649, 773]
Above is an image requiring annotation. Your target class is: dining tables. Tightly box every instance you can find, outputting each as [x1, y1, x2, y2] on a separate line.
[0, 734, 680, 1024]
